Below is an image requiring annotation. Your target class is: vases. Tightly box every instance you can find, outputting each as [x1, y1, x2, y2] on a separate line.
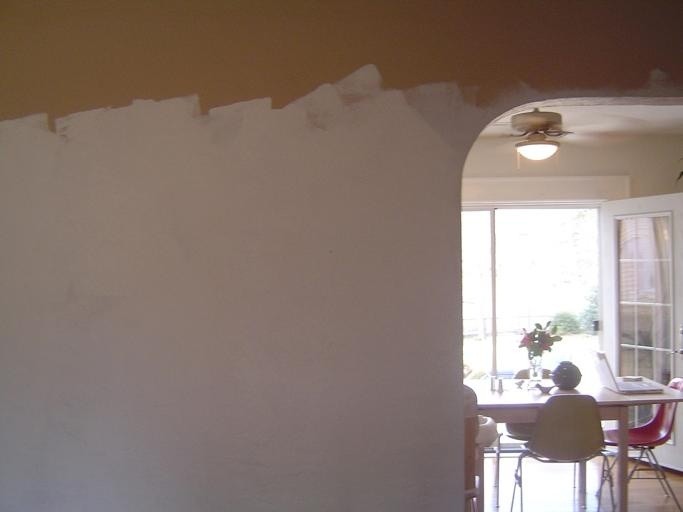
[528, 357, 544, 382]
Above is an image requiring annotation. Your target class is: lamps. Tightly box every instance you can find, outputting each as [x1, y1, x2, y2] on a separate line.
[508, 112, 575, 162]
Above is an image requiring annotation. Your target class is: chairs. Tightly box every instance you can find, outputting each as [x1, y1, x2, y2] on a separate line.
[462, 368, 683, 512]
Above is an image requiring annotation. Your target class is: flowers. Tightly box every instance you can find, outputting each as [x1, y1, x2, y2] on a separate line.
[517, 320, 562, 370]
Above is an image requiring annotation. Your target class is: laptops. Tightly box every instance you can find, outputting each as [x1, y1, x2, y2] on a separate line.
[591, 352, 662, 394]
